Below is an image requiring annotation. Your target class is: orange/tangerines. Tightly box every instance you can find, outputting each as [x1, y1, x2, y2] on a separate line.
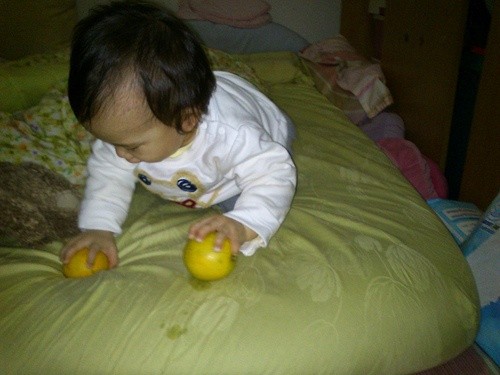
[183, 230, 237, 280]
[63, 247, 109, 278]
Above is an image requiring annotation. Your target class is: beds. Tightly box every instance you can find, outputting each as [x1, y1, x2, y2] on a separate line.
[0, 41, 481, 375]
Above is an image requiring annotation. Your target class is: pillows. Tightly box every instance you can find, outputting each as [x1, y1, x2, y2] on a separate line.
[360, 112, 405, 140]
[424, 198, 482, 245]
[376, 136, 449, 199]
[189, 20, 311, 54]
[458, 194, 500, 374]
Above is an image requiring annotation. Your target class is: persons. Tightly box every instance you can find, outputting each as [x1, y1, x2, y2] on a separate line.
[61, 0, 295, 270]
